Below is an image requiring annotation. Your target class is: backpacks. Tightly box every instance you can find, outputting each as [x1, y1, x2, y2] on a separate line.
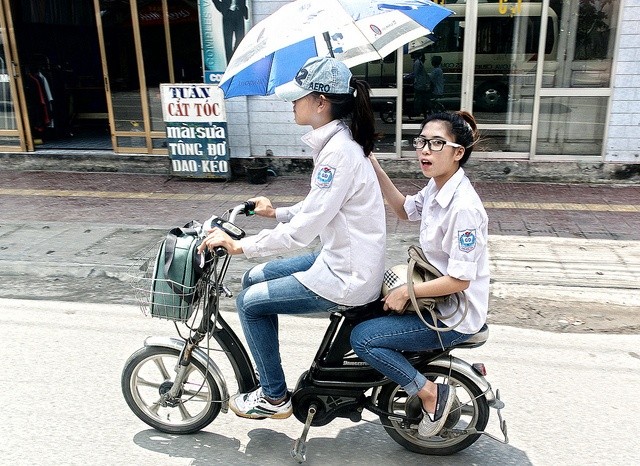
[150, 219, 201, 319]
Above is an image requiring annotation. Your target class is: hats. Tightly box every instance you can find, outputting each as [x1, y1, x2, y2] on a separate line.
[274, 55, 353, 101]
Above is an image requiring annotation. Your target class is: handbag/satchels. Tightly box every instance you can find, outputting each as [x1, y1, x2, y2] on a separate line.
[381, 244, 468, 350]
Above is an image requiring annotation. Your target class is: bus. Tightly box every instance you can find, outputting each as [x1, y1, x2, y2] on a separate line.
[351, 1, 558, 111]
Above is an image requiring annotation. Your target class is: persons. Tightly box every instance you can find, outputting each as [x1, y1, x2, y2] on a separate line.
[211, 0, 248, 66]
[429, 54, 446, 101]
[404, 50, 428, 93]
[195, 56, 388, 419]
[350, 110, 490, 437]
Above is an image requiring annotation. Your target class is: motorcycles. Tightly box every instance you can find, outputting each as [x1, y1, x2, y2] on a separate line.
[381, 76, 445, 124]
[120, 199, 509, 463]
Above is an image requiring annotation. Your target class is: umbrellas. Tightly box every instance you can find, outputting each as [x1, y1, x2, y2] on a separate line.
[216, 0, 456, 99]
[403, 33, 439, 55]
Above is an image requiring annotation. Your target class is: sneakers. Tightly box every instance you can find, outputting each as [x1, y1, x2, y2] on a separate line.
[417, 382, 456, 436]
[228, 386, 292, 418]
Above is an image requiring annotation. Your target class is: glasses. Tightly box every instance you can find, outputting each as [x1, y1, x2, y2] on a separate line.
[411, 136, 460, 151]
